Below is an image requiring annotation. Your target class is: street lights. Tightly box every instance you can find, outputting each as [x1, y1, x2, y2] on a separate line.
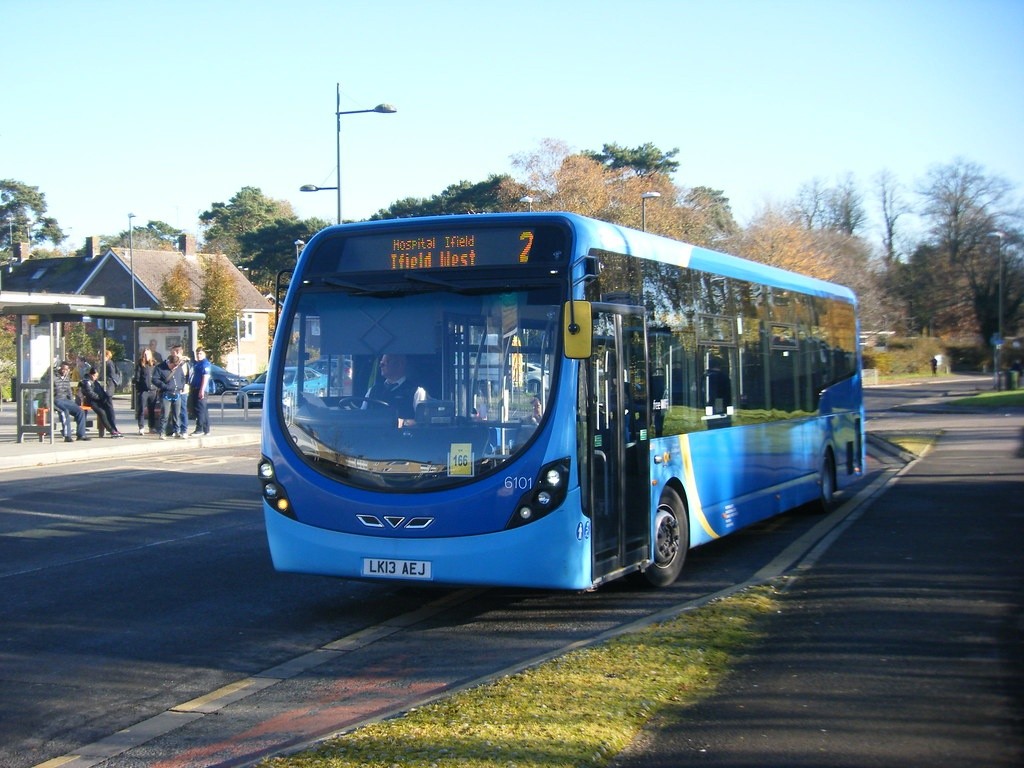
[987, 232, 1003, 392]
[299, 83, 398, 225]
[642, 192, 661, 232]
[128, 213, 136, 310]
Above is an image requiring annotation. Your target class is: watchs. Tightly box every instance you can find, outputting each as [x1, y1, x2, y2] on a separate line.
[403, 419, 409, 427]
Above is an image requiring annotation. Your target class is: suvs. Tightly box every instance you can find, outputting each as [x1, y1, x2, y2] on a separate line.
[306, 359, 353, 386]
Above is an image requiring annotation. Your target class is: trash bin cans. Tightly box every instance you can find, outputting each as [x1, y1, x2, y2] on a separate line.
[1006, 370, 1019, 390]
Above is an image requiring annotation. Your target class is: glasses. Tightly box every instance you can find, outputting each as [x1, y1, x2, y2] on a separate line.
[379, 361, 389, 365]
[197, 353, 203, 355]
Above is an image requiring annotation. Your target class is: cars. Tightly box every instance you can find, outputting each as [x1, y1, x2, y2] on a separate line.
[509, 363, 550, 392]
[236, 366, 328, 409]
[210, 365, 248, 396]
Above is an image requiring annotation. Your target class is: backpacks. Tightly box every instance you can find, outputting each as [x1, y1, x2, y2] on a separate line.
[108, 364, 122, 387]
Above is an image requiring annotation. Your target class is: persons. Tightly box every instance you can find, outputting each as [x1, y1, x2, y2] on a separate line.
[134, 345, 211, 439]
[149, 339, 163, 362]
[43, 345, 125, 442]
[359, 351, 428, 428]
[524, 396, 542, 426]
[930, 357, 937, 374]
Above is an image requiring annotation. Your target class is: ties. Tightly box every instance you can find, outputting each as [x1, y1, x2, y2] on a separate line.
[383, 382, 398, 397]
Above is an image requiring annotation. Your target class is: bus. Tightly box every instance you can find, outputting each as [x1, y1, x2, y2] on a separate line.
[258, 211, 866, 591]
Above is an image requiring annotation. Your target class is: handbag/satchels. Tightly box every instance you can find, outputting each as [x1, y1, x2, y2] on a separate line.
[157, 388, 161, 396]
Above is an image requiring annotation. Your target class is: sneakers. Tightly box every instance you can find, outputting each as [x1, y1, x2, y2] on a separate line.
[65, 437, 73, 442]
[159, 433, 167, 440]
[111, 432, 124, 437]
[176, 434, 183, 439]
[190, 431, 210, 436]
[139, 429, 144, 436]
[182, 433, 188, 439]
[149, 428, 157, 434]
[77, 436, 90, 440]
[172, 433, 176, 437]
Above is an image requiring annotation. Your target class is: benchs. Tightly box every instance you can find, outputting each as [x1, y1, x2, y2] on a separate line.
[36, 406, 107, 443]
